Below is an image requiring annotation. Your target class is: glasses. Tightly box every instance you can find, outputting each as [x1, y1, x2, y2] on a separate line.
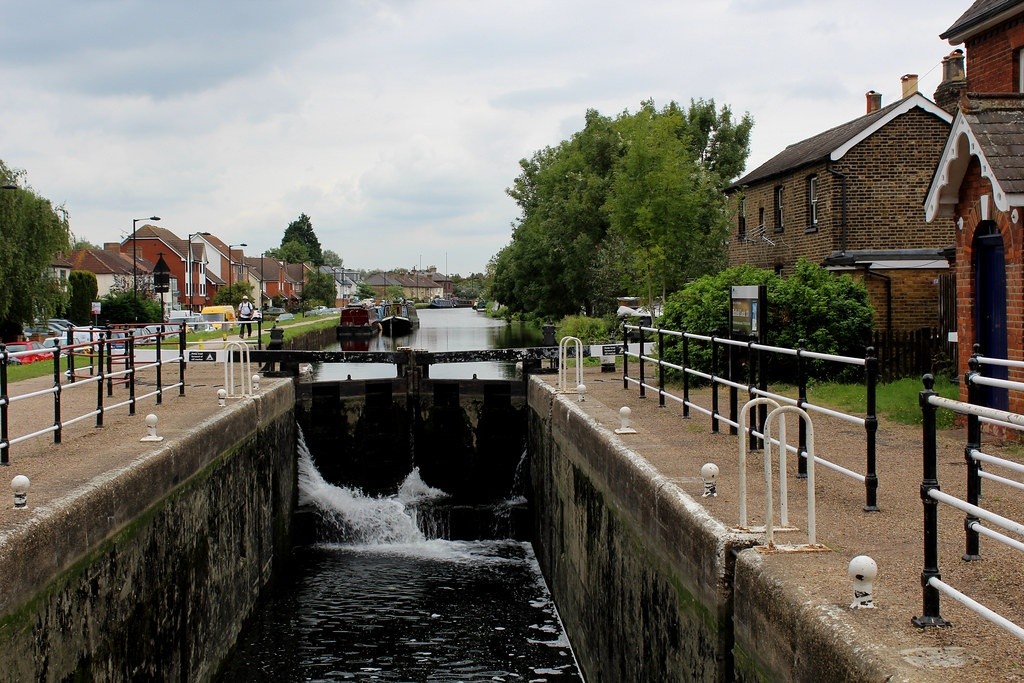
[243, 299, 247, 300]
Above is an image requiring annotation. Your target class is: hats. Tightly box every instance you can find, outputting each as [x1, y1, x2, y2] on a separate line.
[242, 295, 249, 300]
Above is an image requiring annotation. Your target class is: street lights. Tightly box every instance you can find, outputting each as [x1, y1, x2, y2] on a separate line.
[333, 268, 345, 307]
[301, 258, 315, 307]
[227, 243, 248, 305]
[131, 217, 161, 301]
[260, 252, 276, 308]
[317, 263, 330, 304]
[342, 269, 350, 306]
[188, 233, 211, 313]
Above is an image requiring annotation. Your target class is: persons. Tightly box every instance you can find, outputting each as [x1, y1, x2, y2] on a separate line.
[237, 295, 254, 339]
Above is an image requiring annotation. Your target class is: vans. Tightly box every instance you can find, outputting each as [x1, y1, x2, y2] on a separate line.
[200, 305, 237, 331]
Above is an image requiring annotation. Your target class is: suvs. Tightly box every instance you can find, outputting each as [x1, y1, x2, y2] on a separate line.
[263, 307, 287, 316]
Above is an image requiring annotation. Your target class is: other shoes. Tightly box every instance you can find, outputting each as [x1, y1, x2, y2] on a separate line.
[239, 335, 244, 339]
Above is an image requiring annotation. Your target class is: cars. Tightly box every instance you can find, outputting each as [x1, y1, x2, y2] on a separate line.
[276, 313, 295, 324]
[22, 318, 215, 356]
[304, 305, 340, 317]
[251, 310, 263, 323]
[3, 341, 55, 368]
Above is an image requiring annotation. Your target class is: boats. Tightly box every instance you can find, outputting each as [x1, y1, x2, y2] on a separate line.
[338, 302, 383, 338]
[430, 297, 453, 308]
[381, 297, 420, 336]
[454, 299, 475, 307]
